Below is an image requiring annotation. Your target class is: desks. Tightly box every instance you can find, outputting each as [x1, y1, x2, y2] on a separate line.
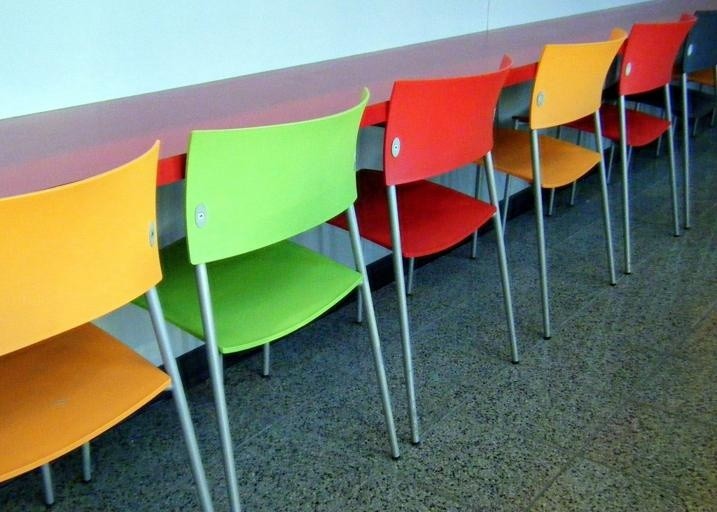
[0, 3, 717, 196]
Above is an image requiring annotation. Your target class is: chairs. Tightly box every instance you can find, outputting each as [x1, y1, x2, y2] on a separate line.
[82, 85, 406, 512]
[0, 136, 215, 512]
[322, 53, 523, 452]
[501, 9, 699, 287]
[470, 23, 631, 341]
[671, 10, 716, 243]
